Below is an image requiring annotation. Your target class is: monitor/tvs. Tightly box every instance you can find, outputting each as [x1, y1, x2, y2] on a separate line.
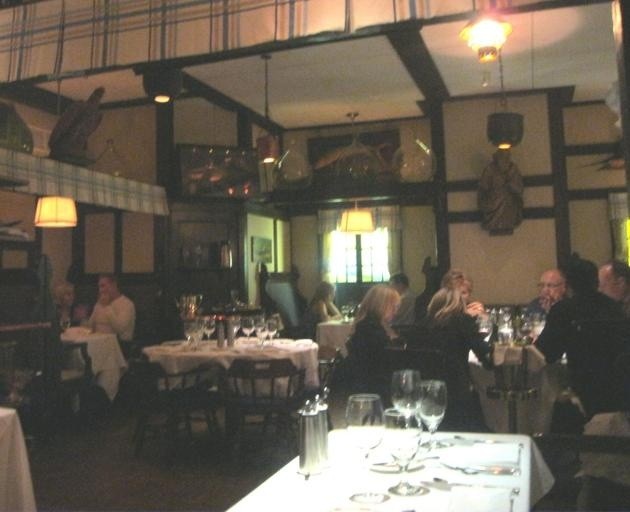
[175, 142, 251, 200]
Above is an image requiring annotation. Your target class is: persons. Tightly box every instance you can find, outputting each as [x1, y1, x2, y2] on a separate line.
[530, 254, 630, 434]
[526, 268, 568, 311]
[410, 272, 496, 433]
[440, 270, 487, 318]
[297, 280, 341, 337]
[344, 285, 403, 396]
[47, 276, 78, 328]
[84, 272, 138, 359]
[385, 270, 419, 326]
[597, 258, 630, 304]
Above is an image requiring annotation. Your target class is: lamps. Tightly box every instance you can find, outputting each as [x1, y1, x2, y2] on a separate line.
[142, 61, 182, 105]
[459, 0, 514, 63]
[34, 78, 81, 228]
[338, 112, 375, 234]
[486, 50, 526, 149]
[256, 53, 277, 163]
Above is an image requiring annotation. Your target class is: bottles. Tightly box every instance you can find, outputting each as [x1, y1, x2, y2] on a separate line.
[295, 387, 329, 477]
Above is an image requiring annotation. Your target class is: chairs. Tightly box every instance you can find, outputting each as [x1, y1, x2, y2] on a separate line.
[1, 321, 332, 465]
[383, 321, 440, 406]
[571, 321, 629, 439]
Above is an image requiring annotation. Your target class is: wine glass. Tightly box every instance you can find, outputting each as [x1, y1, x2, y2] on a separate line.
[344, 365, 448, 504]
[179, 311, 278, 352]
[475, 306, 543, 346]
[341, 303, 360, 323]
[179, 293, 203, 310]
